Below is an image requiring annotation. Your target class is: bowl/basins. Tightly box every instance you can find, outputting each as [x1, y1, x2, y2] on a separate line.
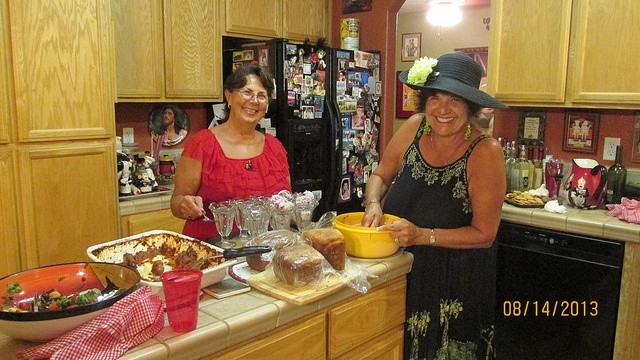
[332, 210, 407, 258]
[1, 261, 142, 344]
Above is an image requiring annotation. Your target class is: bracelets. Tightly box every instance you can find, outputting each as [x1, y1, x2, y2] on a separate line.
[365, 200, 380, 209]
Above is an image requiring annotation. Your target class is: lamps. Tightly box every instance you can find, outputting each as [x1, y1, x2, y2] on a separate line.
[426, 0, 465, 40]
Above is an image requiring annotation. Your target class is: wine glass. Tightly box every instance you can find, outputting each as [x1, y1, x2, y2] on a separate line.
[271, 198, 295, 236]
[208, 200, 236, 252]
[246, 204, 272, 239]
[234, 199, 256, 245]
[293, 199, 320, 236]
[546, 156, 568, 205]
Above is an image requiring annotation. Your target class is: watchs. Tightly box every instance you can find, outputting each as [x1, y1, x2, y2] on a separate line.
[429, 228, 436, 247]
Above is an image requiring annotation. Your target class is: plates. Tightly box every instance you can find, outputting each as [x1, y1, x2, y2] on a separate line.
[229, 261, 264, 284]
[504, 196, 546, 209]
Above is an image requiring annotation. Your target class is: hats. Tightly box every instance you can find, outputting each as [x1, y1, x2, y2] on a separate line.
[398, 51, 506, 109]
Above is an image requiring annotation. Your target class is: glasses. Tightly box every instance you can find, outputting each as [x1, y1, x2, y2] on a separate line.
[229, 88, 271, 104]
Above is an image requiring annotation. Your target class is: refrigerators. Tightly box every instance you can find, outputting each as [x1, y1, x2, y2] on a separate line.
[223, 34, 383, 229]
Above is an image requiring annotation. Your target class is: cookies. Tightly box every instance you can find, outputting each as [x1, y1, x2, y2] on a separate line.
[507, 190, 544, 207]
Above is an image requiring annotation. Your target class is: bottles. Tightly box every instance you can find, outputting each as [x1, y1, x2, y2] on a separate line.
[602, 144, 628, 211]
[504, 141, 511, 162]
[505, 142, 517, 194]
[528, 147, 533, 193]
[534, 148, 546, 189]
[519, 145, 527, 192]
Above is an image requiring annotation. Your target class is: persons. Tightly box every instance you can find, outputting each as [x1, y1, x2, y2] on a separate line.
[341, 179, 350, 201]
[361, 84, 370, 102]
[119, 169, 132, 196]
[170, 63, 296, 241]
[571, 120, 580, 140]
[135, 167, 152, 192]
[348, 155, 358, 172]
[353, 136, 364, 153]
[304, 108, 313, 119]
[581, 120, 591, 140]
[365, 120, 372, 132]
[337, 70, 345, 81]
[364, 173, 369, 182]
[155, 105, 188, 146]
[143, 155, 159, 190]
[362, 133, 370, 152]
[406, 39, 418, 57]
[362, 52, 509, 360]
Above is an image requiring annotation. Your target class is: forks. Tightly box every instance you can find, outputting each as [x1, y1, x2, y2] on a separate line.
[200, 206, 218, 225]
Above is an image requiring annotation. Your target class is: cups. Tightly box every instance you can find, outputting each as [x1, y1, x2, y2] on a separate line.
[547, 164, 562, 198]
[158, 153, 175, 179]
[158, 269, 203, 334]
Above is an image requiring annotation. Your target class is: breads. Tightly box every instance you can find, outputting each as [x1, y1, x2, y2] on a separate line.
[245, 228, 302, 273]
[272, 244, 322, 287]
[303, 226, 347, 273]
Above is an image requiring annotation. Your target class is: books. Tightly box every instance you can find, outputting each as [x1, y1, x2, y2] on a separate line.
[201, 278, 250, 299]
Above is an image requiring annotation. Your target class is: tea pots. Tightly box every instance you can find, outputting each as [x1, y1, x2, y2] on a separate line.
[563, 158, 608, 210]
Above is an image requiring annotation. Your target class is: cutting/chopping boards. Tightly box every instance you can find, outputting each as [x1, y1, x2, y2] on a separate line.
[246, 268, 362, 307]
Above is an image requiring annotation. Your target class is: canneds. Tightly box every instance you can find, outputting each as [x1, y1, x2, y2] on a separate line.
[339, 18, 359, 51]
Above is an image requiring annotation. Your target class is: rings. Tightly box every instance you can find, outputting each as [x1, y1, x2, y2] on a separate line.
[188, 214, 194, 218]
[395, 237, 400, 245]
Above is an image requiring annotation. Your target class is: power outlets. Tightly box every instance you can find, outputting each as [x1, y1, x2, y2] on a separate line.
[603, 136, 620, 161]
[123, 127, 135, 144]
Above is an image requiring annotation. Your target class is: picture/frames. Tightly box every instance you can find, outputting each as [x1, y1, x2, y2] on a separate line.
[630, 110, 640, 163]
[396, 71, 421, 119]
[454, 47, 488, 77]
[342, 0, 373, 14]
[147, 103, 190, 147]
[562, 110, 601, 155]
[401, 32, 421, 62]
[517, 111, 547, 146]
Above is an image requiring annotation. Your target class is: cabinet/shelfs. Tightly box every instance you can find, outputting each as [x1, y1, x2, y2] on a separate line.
[222, 0, 333, 50]
[115, 0, 223, 103]
[200, 309, 327, 360]
[0, 141, 121, 280]
[486, 0, 640, 110]
[612, 239, 640, 360]
[0, 0, 116, 144]
[327, 275, 407, 360]
[119, 209, 187, 238]
